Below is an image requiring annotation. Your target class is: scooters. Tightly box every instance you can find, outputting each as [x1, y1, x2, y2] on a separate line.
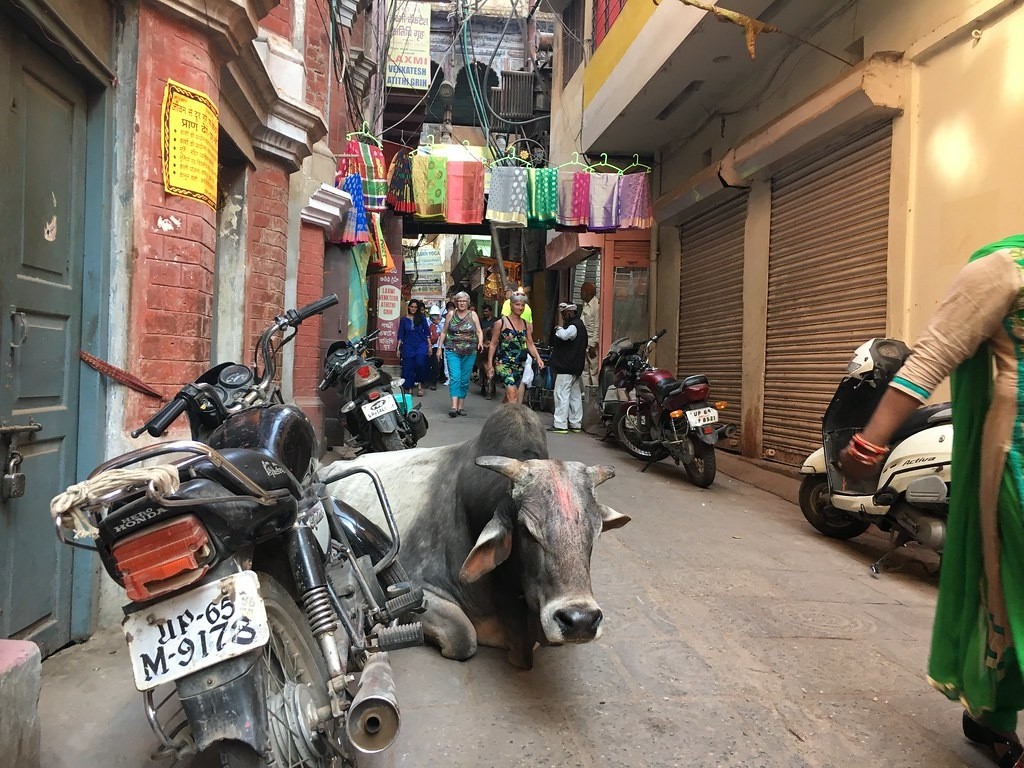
[799, 339, 953, 580]
[597, 338, 654, 442]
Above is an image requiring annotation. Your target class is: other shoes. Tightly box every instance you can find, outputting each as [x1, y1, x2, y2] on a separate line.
[962, 709, 1020, 760]
[546, 425, 569, 433]
[417, 388, 423, 397]
[430, 382, 436, 390]
[448, 408, 458, 417]
[457, 409, 468, 416]
[424, 380, 429, 389]
[569, 427, 581, 433]
[408, 388, 412, 394]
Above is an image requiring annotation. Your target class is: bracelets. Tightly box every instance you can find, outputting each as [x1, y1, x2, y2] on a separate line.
[396, 345, 401, 350]
[847, 434, 890, 467]
[488, 368, 491, 371]
[438, 346, 442, 348]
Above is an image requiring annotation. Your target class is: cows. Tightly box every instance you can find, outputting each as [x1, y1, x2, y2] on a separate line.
[312, 403, 632, 671]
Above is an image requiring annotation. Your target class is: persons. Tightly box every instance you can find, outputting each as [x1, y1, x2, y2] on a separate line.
[406, 300, 476, 390]
[486, 295, 545, 406]
[396, 299, 433, 397]
[545, 302, 588, 434]
[479, 305, 499, 400]
[579, 283, 600, 398]
[501, 282, 533, 404]
[836, 234, 1024, 768]
[437, 291, 484, 418]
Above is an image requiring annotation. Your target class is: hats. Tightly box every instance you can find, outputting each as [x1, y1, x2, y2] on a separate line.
[429, 305, 440, 314]
[559, 302, 578, 311]
[583, 282, 596, 294]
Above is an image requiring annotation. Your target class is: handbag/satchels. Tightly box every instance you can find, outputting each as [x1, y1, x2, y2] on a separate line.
[519, 350, 529, 362]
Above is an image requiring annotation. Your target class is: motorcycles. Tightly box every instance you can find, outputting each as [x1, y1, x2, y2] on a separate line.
[49, 292, 427, 768]
[317, 326, 429, 457]
[615, 328, 738, 488]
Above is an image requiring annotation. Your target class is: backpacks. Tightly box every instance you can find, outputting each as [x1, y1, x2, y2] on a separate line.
[430, 323, 438, 343]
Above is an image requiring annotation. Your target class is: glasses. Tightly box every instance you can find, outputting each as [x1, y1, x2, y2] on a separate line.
[510, 292, 527, 300]
[503, 290, 512, 295]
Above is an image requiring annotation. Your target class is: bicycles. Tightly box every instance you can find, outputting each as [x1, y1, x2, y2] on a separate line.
[526, 341, 554, 411]
